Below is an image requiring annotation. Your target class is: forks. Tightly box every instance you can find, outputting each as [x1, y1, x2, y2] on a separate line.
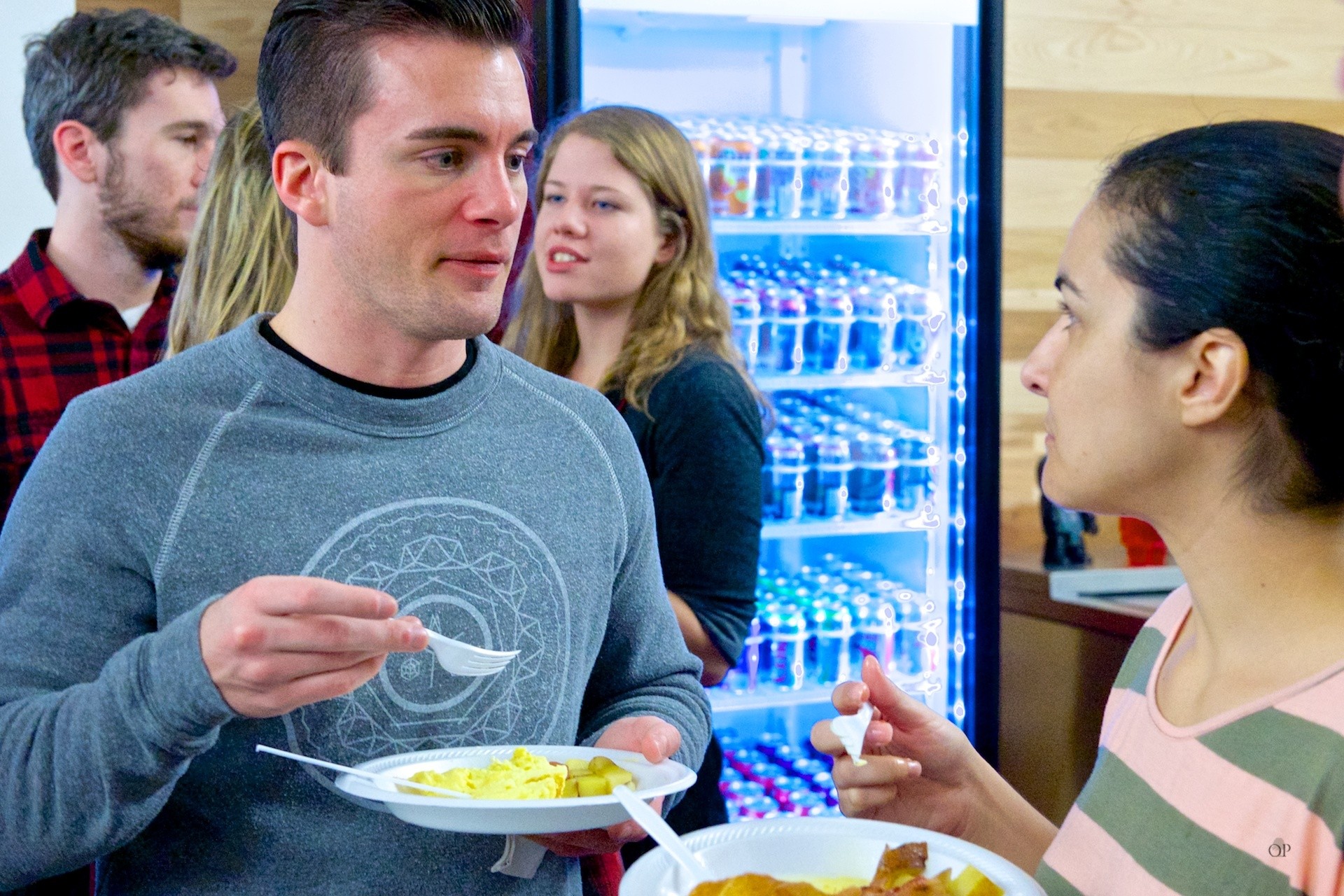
[833, 701, 874, 769]
[430, 628, 521, 681]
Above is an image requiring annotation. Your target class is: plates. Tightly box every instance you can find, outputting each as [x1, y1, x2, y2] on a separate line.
[334, 745, 697, 835]
[617, 815, 1052, 896]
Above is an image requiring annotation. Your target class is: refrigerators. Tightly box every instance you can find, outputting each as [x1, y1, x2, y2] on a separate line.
[533, 0, 1004, 828]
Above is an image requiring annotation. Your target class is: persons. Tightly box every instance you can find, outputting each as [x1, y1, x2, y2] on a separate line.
[1, 10, 227, 530]
[0, 2, 708, 896]
[500, 103, 771, 864]
[163, 90, 299, 370]
[810, 116, 1344, 896]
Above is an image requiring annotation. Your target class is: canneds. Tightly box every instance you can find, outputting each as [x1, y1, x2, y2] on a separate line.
[642, 110, 947, 826]
